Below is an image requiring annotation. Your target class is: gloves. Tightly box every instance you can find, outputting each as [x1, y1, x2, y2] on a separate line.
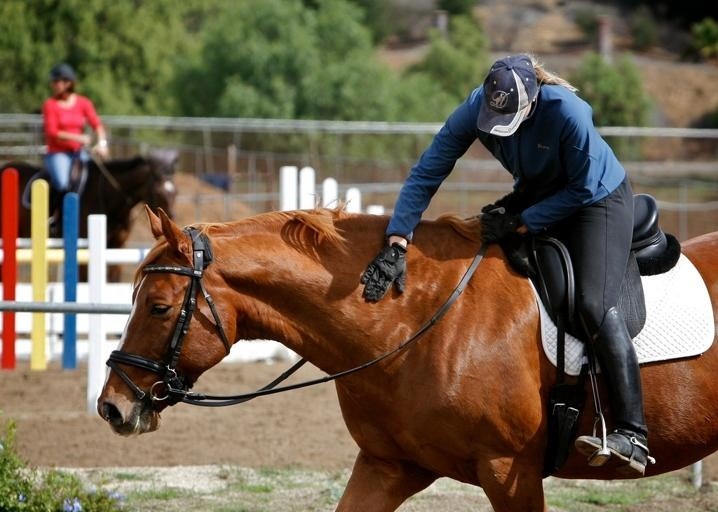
[479, 204, 523, 242]
[361, 235, 408, 302]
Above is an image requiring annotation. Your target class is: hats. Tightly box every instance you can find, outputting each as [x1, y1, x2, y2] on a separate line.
[476, 54, 538, 138]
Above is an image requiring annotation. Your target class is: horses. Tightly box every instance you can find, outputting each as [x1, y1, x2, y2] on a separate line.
[96, 203, 718, 512]
[0, 151, 182, 283]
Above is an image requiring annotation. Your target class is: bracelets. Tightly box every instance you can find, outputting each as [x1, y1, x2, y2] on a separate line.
[95, 139, 110, 150]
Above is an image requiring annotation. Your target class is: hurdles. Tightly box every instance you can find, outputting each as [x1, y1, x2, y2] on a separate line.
[29, 166, 317, 368]
[87, 186, 362, 413]
[62, 177, 339, 371]
[1, 166, 302, 372]
[367, 204, 384, 218]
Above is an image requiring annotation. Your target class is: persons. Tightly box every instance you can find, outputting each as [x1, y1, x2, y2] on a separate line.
[358, 53, 657, 479]
[41, 62, 114, 229]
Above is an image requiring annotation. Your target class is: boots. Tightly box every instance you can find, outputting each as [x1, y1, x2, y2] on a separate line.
[575, 306, 648, 479]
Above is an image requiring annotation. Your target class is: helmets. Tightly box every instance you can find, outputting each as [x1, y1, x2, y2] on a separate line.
[48, 63, 75, 82]
